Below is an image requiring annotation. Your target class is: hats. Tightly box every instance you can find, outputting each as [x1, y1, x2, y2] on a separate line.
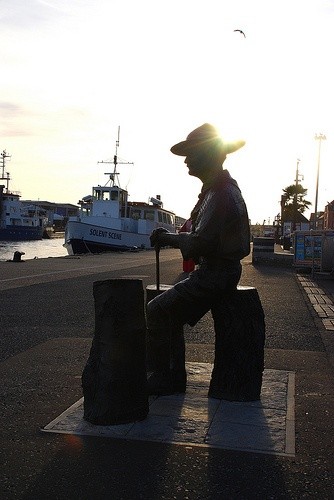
[170, 123, 246, 154]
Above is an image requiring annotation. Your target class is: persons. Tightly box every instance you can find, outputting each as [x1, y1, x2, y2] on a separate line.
[144, 123, 252, 393]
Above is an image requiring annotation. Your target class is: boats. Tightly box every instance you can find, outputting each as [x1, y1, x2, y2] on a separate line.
[62, 125, 176, 255]
[0, 151, 44, 240]
